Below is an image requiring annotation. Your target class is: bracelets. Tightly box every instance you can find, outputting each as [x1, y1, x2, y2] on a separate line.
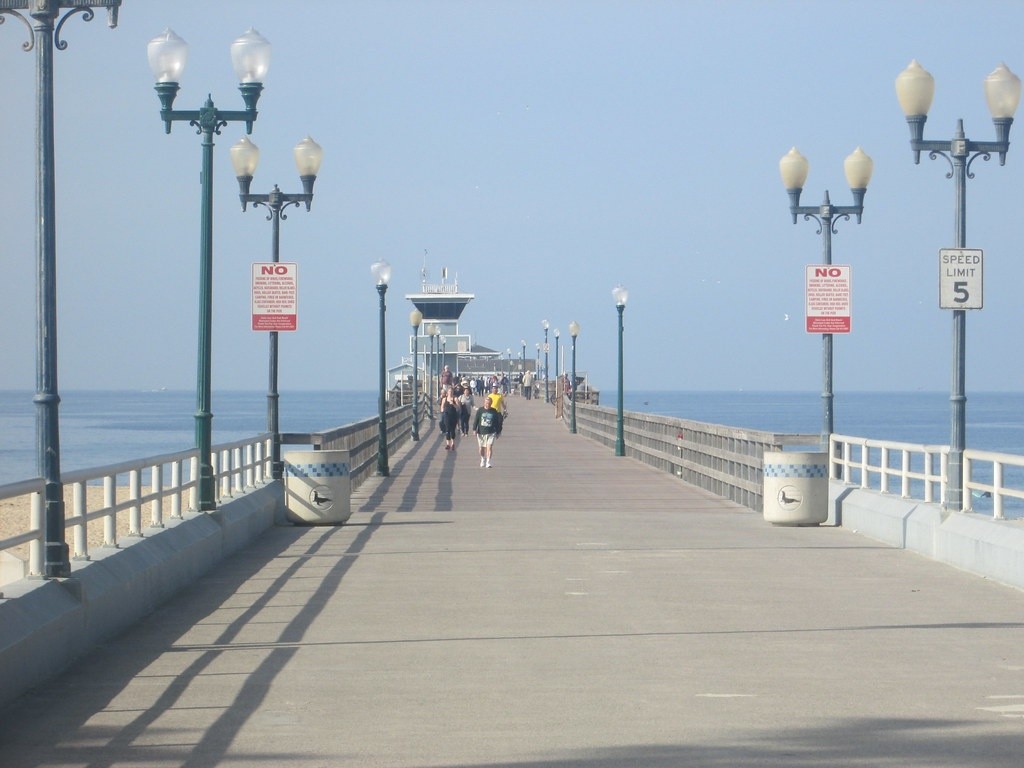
[504, 409, 507, 412]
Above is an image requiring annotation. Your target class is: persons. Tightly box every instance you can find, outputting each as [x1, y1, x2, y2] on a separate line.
[457, 388, 476, 437]
[484, 375, 498, 394]
[440, 387, 461, 451]
[487, 383, 507, 424]
[514, 369, 533, 400]
[473, 397, 500, 468]
[437, 389, 447, 434]
[564, 373, 570, 393]
[440, 364, 452, 392]
[499, 373, 509, 397]
[451, 373, 484, 397]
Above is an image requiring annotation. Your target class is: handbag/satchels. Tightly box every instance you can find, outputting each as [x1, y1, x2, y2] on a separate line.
[439, 421, 446, 433]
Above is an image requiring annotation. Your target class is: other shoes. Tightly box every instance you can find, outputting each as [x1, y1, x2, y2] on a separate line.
[486, 461, 492, 469]
[480, 458, 485, 467]
[451, 445, 455, 450]
[445, 445, 449, 449]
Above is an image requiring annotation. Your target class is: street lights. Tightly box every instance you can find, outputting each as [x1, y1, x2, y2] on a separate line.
[775, 143, 876, 479]
[517, 352, 523, 398]
[439, 335, 447, 371]
[567, 320, 580, 435]
[535, 342, 542, 380]
[520, 339, 528, 376]
[140, 23, 273, 513]
[612, 282, 629, 457]
[539, 318, 551, 403]
[509, 360, 514, 382]
[552, 328, 561, 377]
[891, 57, 1024, 512]
[506, 347, 513, 394]
[369, 258, 393, 476]
[434, 325, 443, 406]
[427, 321, 437, 420]
[227, 134, 326, 481]
[408, 307, 423, 442]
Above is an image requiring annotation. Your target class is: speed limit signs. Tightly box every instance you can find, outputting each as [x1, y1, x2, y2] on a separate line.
[936, 248, 985, 312]
